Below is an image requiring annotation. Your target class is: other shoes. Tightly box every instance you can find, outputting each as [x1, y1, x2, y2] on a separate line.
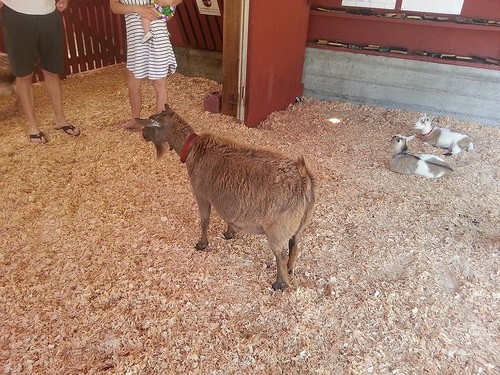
[141, 31, 153, 44]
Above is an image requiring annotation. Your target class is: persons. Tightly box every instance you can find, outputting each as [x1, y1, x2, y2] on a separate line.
[1, 0, 80, 144]
[109, 0, 181, 129]
[141, 0, 181, 43]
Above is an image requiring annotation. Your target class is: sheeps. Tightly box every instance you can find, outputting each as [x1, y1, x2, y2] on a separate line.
[415, 112, 473, 156]
[389, 134, 454, 179]
[133, 102, 319, 292]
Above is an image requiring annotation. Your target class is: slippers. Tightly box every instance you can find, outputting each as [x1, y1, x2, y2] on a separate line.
[55, 124, 81, 136]
[30, 132, 48, 144]
